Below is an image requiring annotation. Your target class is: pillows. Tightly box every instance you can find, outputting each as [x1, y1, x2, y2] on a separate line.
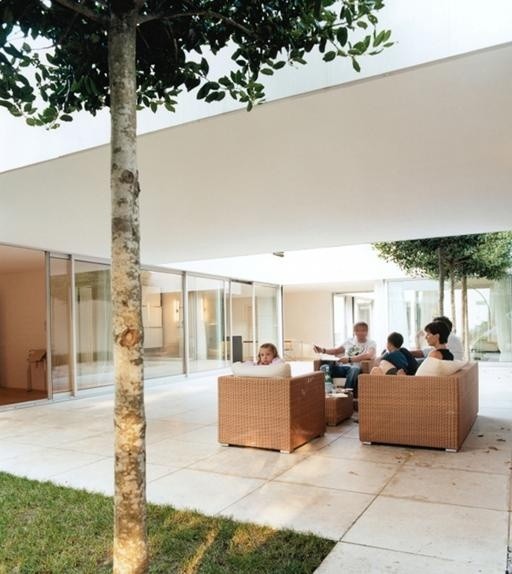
[415, 356, 465, 376]
[233, 362, 291, 378]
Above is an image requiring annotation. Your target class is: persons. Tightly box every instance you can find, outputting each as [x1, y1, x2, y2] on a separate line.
[409, 315, 463, 361]
[370, 321, 453, 375]
[381, 332, 418, 376]
[312, 321, 377, 389]
[242, 342, 285, 367]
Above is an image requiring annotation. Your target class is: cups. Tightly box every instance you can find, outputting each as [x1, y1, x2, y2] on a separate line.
[332, 384, 337, 395]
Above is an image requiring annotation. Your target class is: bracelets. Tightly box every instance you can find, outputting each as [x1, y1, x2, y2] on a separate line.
[348, 357, 352, 364]
[323, 348, 326, 355]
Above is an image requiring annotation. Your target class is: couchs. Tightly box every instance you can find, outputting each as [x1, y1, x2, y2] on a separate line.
[218, 371, 325, 453]
[314, 360, 375, 411]
[357, 358, 478, 453]
